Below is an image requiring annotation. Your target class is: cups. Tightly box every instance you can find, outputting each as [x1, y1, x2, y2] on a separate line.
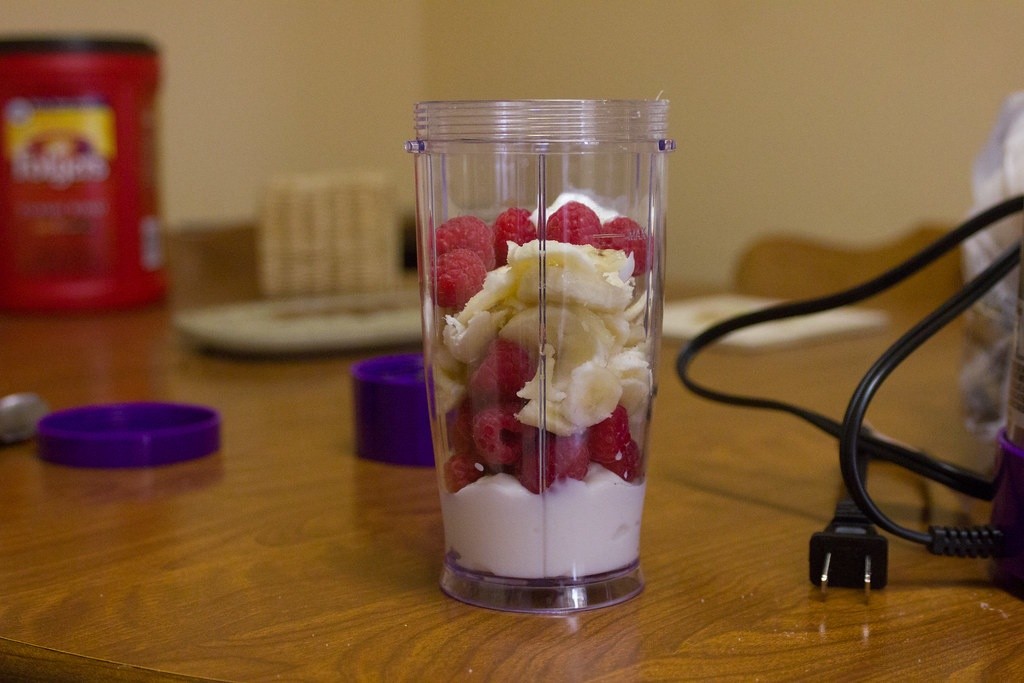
[403, 98, 676, 615]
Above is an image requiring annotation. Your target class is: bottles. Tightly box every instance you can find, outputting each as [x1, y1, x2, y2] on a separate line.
[0, 32, 171, 317]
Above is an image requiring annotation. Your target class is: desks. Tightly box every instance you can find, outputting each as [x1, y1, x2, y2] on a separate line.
[0, 290, 1024, 683]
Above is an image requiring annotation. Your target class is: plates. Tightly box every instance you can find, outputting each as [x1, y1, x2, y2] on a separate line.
[167, 291, 423, 352]
